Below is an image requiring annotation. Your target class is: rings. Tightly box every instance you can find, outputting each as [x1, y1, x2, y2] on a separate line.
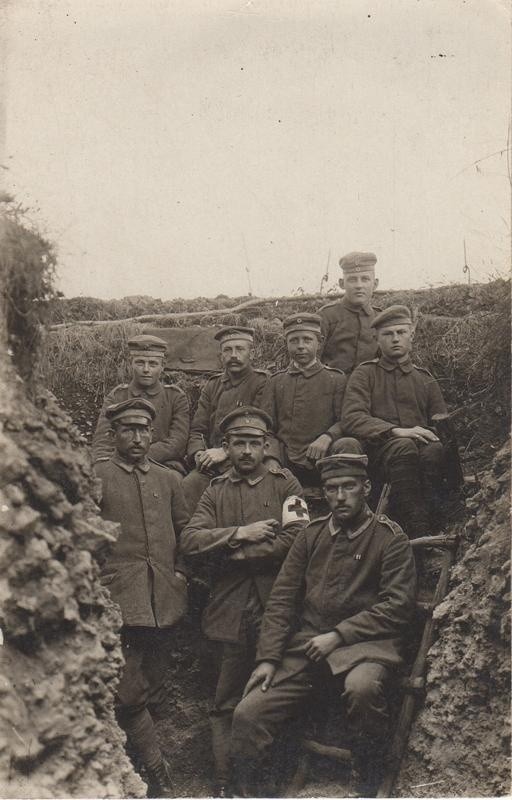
[415, 434, 422, 440]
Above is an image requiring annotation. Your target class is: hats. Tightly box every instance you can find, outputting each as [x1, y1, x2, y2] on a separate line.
[339, 251, 378, 274]
[127, 335, 168, 358]
[314, 453, 369, 483]
[281, 312, 323, 336]
[217, 405, 273, 439]
[212, 325, 255, 348]
[367, 304, 413, 329]
[105, 397, 157, 424]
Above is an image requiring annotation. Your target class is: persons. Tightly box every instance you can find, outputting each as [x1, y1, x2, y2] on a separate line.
[180, 327, 275, 517]
[82, 395, 200, 798]
[335, 304, 460, 553]
[213, 445, 423, 797]
[254, 312, 347, 488]
[314, 250, 389, 369]
[178, 405, 315, 797]
[87, 329, 190, 481]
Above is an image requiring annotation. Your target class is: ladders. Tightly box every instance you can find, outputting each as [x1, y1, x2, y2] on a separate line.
[283, 482, 463, 800]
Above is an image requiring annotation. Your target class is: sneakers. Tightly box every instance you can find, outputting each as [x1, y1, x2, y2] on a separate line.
[217, 777, 266, 797]
[409, 527, 435, 553]
[152, 772, 175, 798]
